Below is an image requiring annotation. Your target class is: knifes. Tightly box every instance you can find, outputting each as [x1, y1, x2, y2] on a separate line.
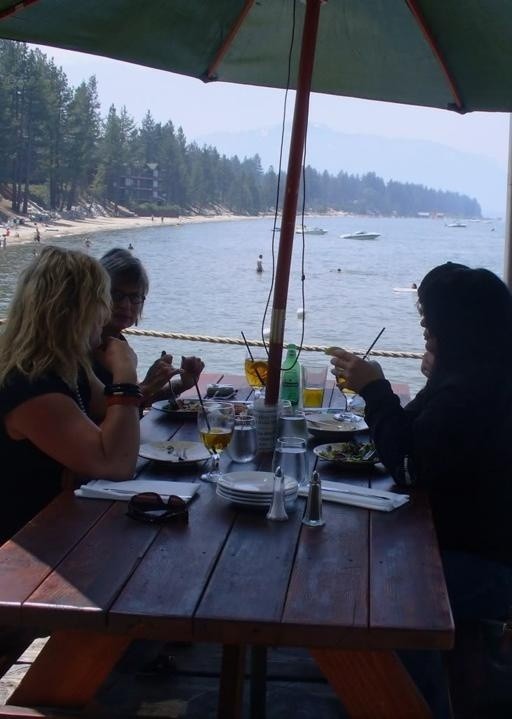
[305, 484, 391, 501]
[102, 487, 193, 500]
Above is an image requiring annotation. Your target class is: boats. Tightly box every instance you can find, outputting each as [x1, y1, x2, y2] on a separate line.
[471, 216, 482, 224]
[446, 220, 467, 230]
[341, 229, 384, 245]
[390, 278, 423, 297]
[295, 226, 325, 236]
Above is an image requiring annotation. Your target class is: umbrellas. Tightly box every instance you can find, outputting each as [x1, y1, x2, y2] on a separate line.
[1, 0, 512, 470]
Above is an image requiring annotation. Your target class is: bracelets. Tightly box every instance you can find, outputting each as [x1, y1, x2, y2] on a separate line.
[103, 382, 146, 408]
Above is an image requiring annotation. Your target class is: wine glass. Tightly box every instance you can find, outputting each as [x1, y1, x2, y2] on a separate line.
[197, 350, 370, 487]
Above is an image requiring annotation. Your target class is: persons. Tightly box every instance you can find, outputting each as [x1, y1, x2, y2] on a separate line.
[3, 217, 41, 259]
[2, 245, 175, 681]
[79, 248, 204, 426]
[150, 213, 183, 226]
[128, 241, 133, 252]
[84, 236, 92, 247]
[410, 282, 418, 289]
[256, 252, 264, 274]
[325, 261, 512, 719]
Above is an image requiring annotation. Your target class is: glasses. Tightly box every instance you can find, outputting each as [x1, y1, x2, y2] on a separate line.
[125, 493, 200, 524]
[111, 290, 145, 304]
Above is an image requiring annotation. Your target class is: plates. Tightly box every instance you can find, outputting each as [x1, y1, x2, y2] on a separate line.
[137, 398, 383, 507]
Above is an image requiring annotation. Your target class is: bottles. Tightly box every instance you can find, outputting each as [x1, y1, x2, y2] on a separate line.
[280, 344, 301, 406]
[265, 465, 326, 527]
